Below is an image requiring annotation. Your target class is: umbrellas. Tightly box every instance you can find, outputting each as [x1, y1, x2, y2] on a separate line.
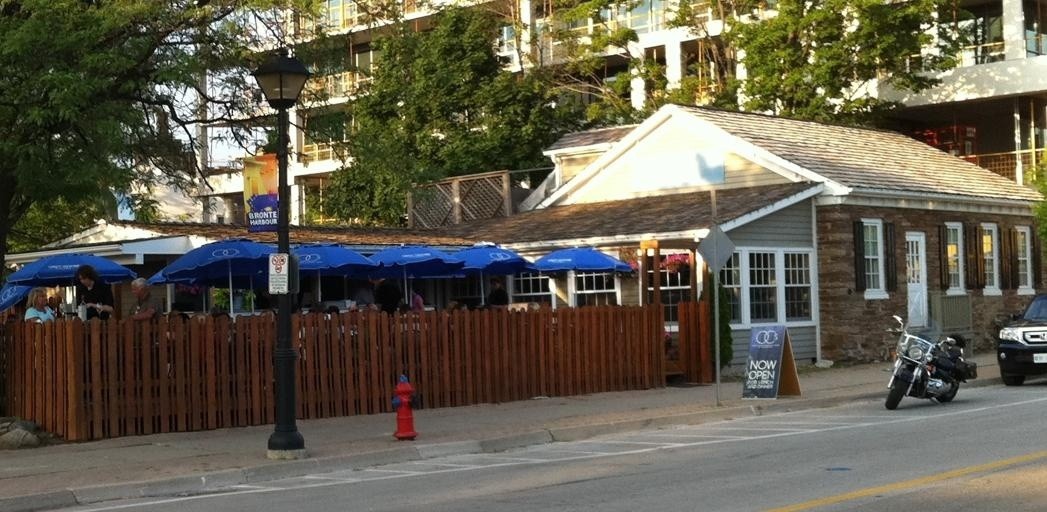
[366, 241, 466, 303]
[162, 236, 279, 313]
[5, 250, 136, 309]
[145, 266, 193, 314]
[198, 273, 269, 311]
[525, 245, 633, 306]
[291, 240, 375, 302]
[0, 282, 37, 313]
[449, 243, 534, 303]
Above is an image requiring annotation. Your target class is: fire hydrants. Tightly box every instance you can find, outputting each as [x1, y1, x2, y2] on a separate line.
[392, 374, 419, 441]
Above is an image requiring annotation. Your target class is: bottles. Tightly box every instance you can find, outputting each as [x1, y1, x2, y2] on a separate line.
[78, 295, 88, 320]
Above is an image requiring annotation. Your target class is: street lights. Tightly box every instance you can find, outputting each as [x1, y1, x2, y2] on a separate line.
[253, 47, 312, 460]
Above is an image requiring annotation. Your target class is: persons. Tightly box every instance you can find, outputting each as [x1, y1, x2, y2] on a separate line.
[75, 264, 114, 319]
[23, 287, 58, 322]
[204, 288, 410, 325]
[485, 275, 508, 306]
[127, 278, 163, 320]
[408, 286, 424, 312]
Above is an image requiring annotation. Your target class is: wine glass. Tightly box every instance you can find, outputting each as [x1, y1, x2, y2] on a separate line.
[96, 302, 103, 319]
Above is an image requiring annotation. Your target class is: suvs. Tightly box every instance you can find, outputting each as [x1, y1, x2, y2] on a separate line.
[998, 292, 1047, 387]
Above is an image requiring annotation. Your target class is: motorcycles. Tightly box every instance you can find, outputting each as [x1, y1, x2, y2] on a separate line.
[884, 312, 977, 411]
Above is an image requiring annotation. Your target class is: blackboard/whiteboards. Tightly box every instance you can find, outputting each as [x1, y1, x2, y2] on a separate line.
[743, 325, 785, 401]
